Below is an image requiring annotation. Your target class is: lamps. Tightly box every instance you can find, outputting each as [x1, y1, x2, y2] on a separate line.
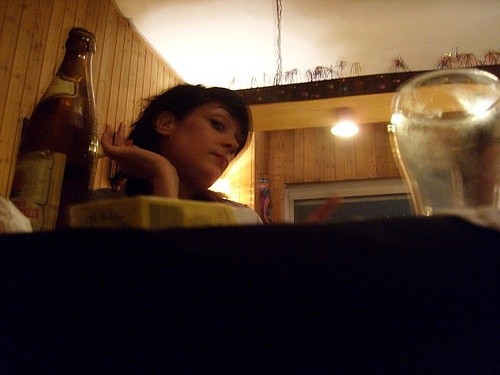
[327, 106, 361, 138]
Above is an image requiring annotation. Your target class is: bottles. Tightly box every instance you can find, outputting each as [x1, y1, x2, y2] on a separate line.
[7, 23, 100, 233]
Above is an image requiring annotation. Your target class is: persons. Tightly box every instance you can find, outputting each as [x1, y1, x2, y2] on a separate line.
[101, 83, 254, 203]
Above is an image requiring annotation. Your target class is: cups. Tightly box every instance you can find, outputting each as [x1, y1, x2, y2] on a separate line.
[387, 63, 500, 232]
